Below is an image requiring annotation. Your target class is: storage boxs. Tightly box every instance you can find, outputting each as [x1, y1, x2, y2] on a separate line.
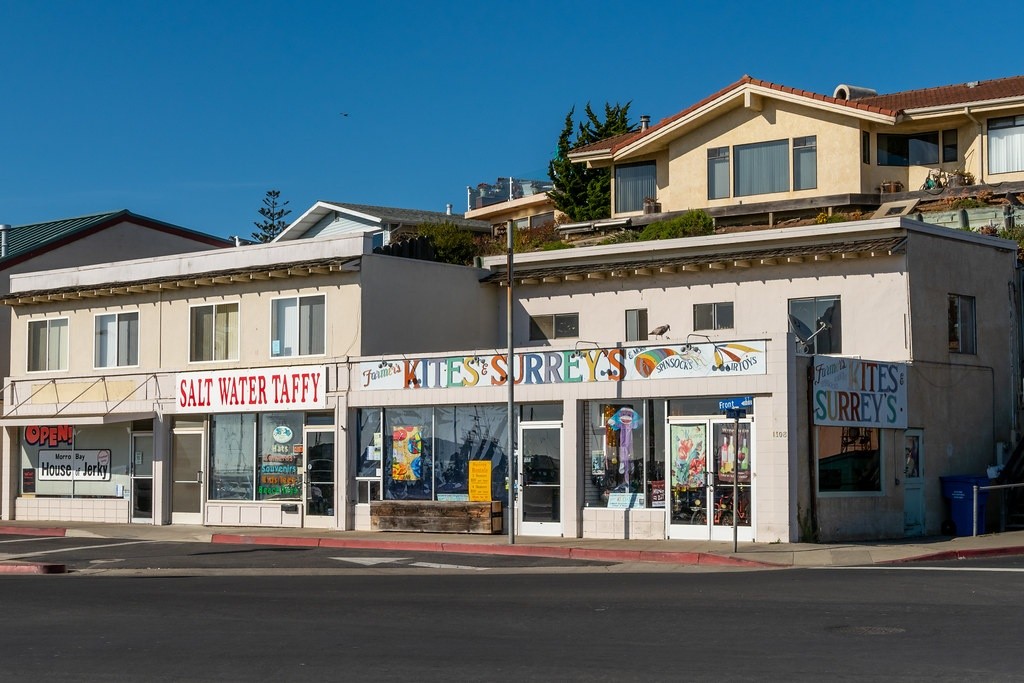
[370, 500, 504, 536]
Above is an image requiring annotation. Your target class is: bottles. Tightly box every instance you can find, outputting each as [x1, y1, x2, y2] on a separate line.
[720, 436, 749, 473]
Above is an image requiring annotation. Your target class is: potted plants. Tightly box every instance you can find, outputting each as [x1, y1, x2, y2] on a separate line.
[981, 222, 1001, 236]
[642, 196, 662, 214]
[881, 181, 904, 193]
[947, 169, 977, 187]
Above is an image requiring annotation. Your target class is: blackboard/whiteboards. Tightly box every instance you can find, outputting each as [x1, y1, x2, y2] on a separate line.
[22, 467, 37, 494]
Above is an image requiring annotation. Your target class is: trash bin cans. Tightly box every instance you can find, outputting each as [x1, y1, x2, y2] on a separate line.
[937, 473, 991, 536]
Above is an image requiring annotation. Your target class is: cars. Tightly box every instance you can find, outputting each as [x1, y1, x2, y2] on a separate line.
[520, 454, 557, 483]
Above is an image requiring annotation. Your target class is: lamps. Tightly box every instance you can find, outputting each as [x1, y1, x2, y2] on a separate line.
[570, 341, 611, 360]
[378, 352, 416, 372]
[680, 333, 715, 352]
[470, 347, 508, 367]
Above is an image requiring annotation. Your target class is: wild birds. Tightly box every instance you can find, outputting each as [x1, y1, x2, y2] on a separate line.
[338, 112, 352, 119]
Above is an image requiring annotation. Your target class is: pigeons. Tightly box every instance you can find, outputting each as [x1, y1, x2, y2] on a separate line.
[648, 323, 671, 339]
